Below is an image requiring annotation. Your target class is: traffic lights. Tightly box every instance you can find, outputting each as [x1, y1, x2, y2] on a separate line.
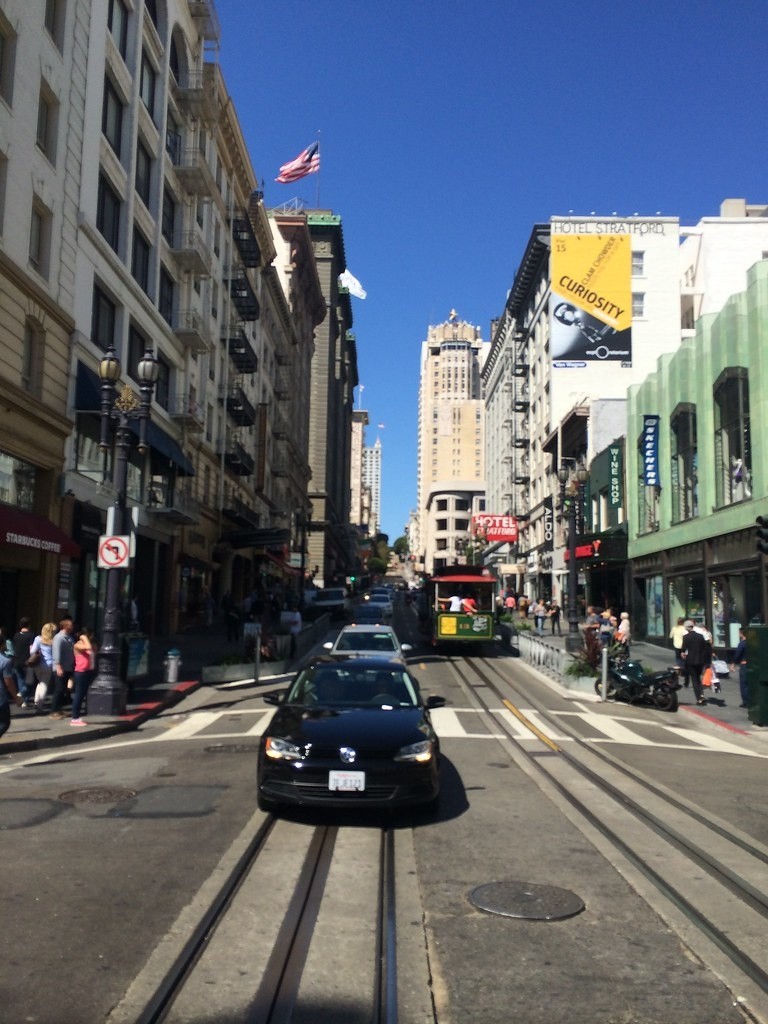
[755, 515, 768, 556]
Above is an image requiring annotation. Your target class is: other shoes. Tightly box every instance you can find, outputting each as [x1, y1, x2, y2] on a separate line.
[48, 713, 63, 720]
[739, 703, 746, 707]
[69, 719, 87, 726]
[695, 697, 706, 706]
[20, 701, 26, 707]
[684, 683, 688, 689]
[61, 709, 72, 717]
[17, 692, 22, 697]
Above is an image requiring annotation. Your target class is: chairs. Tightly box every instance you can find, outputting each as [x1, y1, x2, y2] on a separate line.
[370, 671, 402, 700]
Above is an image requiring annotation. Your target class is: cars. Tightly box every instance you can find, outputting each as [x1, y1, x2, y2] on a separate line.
[323, 623, 412, 670]
[349, 604, 387, 626]
[360, 594, 396, 619]
[362, 583, 406, 607]
[256, 655, 447, 816]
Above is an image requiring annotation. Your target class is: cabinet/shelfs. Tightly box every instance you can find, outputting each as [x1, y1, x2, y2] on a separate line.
[715, 623, 726, 647]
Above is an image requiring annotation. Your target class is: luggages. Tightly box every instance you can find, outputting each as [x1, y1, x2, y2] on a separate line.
[712, 654, 730, 677]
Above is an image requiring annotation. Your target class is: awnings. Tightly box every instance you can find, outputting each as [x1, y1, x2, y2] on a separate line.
[76, 363, 197, 477]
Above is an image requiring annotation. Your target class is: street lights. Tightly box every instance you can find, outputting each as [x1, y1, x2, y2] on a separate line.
[84, 345, 157, 725]
[556, 461, 592, 654]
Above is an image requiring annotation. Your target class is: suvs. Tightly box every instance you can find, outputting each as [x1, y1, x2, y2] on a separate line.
[308, 586, 352, 622]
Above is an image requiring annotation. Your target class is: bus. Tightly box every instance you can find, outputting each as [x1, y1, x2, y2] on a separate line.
[416, 565, 500, 650]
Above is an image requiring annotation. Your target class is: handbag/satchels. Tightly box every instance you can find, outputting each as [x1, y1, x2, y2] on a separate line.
[702, 668, 713, 686]
[710, 669, 721, 694]
[25, 649, 42, 667]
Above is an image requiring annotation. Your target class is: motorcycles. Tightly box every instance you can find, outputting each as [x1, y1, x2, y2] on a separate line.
[404, 592, 413, 608]
[593, 635, 686, 713]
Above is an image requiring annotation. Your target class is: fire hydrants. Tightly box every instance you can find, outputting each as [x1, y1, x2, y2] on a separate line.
[162, 647, 184, 683]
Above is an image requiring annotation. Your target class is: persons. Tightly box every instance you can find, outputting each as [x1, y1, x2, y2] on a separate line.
[462, 593, 477, 612]
[223, 588, 265, 642]
[49, 620, 75, 721]
[70, 627, 95, 728]
[203, 592, 217, 627]
[286, 604, 303, 660]
[0, 616, 58, 738]
[437, 591, 478, 613]
[494, 584, 768, 728]
